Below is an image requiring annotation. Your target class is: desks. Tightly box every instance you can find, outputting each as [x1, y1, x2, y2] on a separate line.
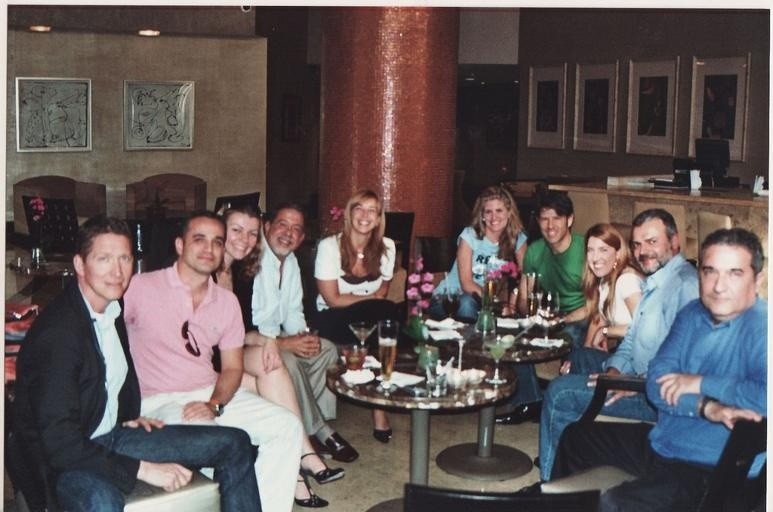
[435, 329, 575, 482]
[326, 363, 520, 510]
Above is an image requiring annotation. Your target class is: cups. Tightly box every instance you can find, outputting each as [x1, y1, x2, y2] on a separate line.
[690, 168, 702, 191]
[425, 360, 449, 397]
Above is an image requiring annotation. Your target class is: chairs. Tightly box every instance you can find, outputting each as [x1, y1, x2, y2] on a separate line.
[7, 174, 767, 369]
[547, 368, 766, 509]
[6, 381, 220, 512]
[403, 481, 602, 511]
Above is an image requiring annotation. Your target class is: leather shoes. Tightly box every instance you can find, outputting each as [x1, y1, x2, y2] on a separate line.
[495, 401, 542, 424]
[374, 428, 392, 443]
[309, 433, 358, 461]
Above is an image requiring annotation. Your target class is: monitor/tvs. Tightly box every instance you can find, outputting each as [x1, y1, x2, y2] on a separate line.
[694, 138, 730, 183]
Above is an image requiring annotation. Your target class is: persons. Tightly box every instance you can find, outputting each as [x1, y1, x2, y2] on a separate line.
[429, 186, 529, 320]
[559, 223, 646, 376]
[304, 188, 398, 442]
[211, 200, 344, 507]
[494, 191, 602, 424]
[251, 201, 360, 461]
[14, 217, 263, 511]
[122, 210, 304, 511]
[548, 228, 767, 512]
[534, 208, 702, 481]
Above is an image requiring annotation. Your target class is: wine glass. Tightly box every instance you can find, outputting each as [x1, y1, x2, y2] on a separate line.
[443, 288, 461, 318]
[485, 334, 506, 384]
[338, 322, 378, 368]
[379, 321, 400, 393]
[540, 291, 560, 343]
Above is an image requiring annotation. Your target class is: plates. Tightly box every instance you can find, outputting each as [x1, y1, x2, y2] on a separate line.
[529, 337, 565, 348]
[376, 371, 427, 388]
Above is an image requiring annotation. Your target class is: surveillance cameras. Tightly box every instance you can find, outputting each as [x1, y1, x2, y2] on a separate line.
[241, 6, 252, 14]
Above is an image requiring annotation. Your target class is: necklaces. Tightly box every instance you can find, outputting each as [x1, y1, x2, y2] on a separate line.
[356, 252, 366, 258]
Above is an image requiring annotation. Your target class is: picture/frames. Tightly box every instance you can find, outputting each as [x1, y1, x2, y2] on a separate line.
[15, 76, 93, 154]
[526, 53, 750, 163]
[122, 79, 196, 152]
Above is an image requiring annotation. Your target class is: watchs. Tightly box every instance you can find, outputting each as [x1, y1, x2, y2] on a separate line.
[511, 287, 520, 298]
[208, 398, 225, 417]
[602, 323, 611, 336]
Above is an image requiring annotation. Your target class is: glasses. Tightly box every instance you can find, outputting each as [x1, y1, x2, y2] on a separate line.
[182, 321, 201, 357]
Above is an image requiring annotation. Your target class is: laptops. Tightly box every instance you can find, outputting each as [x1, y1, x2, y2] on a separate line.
[648, 157, 696, 187]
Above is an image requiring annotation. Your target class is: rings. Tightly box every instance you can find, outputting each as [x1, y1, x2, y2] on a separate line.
[566, 369, 570, 373]
[560, 365, 564, 369]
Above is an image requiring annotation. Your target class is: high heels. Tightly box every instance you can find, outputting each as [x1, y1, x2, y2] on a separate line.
[295, 481, 329, 507]
[298, 453, 345, 490]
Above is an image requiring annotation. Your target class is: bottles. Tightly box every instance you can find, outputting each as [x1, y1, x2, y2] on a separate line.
[475, 278, 497, 335]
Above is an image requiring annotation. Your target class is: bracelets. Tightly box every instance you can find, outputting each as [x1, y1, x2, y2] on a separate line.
[557, 314, 567, 327]
[700, 396, 716, 418]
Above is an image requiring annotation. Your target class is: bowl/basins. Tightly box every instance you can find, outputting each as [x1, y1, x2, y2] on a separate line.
[460, 368, 485, 385]
